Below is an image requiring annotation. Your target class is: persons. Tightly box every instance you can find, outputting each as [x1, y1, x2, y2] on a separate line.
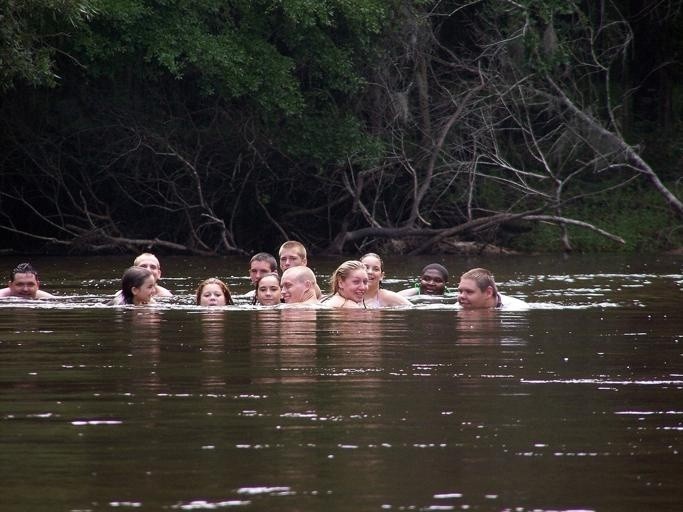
[194, 277, 234, 306]
[277, 240, 321, 301]
[279, 265, 318, 305]
[243, 252, 278, 294]
[359, 252, 414, 308]
[107, 267, 157, 306]
[249, 272, 284, 306]
[0, 262, 55, 299]
[317, 260, 369, 309]
[451, 268, 526, 310]
[397, 263, 459, 298]
[113, 253, 172, 297]
